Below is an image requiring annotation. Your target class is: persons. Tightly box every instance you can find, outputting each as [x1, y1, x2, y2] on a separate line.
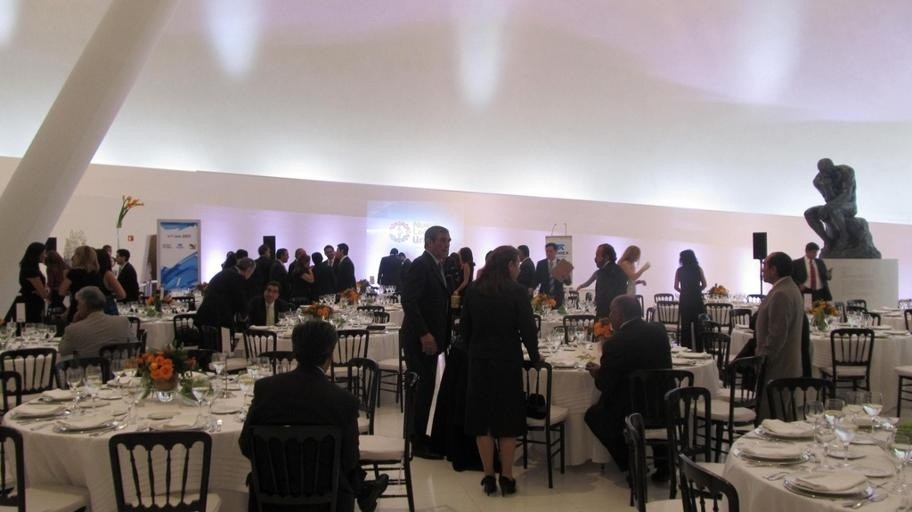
[805, 158, 865, 256]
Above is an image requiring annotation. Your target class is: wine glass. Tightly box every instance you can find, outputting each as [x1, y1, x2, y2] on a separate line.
[539, 323, 592, 358]
[116, 301, 189, 320]
[274, 304, 389, 332]
[881, 432, 912, 498]
[862, 389, 884, 435]
[212, 353, 229, 393]
[320, 283, 397, 305]
[817, 303, 873, 329]
[805, 395, 855, 471]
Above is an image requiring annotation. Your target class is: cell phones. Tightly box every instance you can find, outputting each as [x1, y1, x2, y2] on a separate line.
[828, 267, 833, 272]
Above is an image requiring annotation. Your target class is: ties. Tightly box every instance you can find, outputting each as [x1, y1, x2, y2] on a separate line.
[329, 259, 332, 268]
[549, 261, 555, 293]
[809, 260, 816, 289]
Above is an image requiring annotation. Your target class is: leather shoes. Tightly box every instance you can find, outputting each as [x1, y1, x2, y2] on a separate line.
[626, 465, 649, 488]
[652, 467, 670, 481]
[357, 474, 389, 511]
[412, 441, 444, 459]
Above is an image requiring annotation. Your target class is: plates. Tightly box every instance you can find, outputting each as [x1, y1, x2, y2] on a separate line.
[669, 346, 711, 369]
[15, 356, 291, 435]
[741, 321, 909, 341]
[732, 417, 892, 498]
[550, 360, 580, 370]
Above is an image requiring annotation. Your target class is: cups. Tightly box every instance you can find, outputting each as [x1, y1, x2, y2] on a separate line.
[0, 320, 57, 351]
[702, 293, 761, 306]
[544, 299, 595, 322]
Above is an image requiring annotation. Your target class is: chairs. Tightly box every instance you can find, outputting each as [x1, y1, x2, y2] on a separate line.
[0, 280, 912, 512]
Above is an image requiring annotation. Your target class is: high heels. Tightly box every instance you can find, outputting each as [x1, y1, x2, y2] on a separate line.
[499, 473, 516, 496]
[481, 474, 496, 496]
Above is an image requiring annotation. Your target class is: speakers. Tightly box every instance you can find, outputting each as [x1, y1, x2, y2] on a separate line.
[753, 232, 767, 259]
[263, 236, 276, 257]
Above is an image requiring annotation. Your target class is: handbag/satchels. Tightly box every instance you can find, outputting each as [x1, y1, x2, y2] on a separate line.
[526, 393, 546, 419]
